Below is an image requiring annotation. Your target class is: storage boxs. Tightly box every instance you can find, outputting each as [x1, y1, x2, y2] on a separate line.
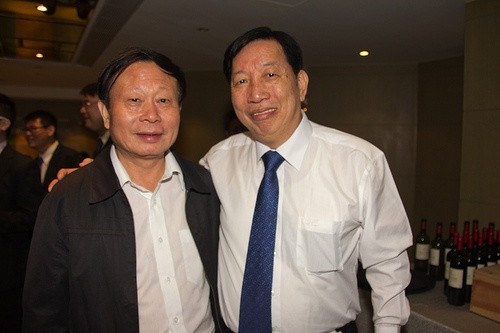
[469, 265, 500, 322]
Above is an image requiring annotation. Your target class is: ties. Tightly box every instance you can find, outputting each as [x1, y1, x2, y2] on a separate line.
[40, 155, 51, 182]
[238, 150, 284, 333]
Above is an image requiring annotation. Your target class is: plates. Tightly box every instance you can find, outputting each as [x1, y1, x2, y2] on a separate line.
[405, 269, 436, 294]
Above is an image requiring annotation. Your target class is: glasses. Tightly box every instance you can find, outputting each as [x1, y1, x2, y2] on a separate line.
[80, 100, 97, 108]
[24, 126, 43, 132]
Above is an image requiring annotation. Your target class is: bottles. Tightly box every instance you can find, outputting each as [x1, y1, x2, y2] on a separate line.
[430, 222, 444, 281]
[445, 219, 500, 306]
[414, 218, 430, 273]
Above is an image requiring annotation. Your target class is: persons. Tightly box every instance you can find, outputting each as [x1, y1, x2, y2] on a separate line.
[48, 26, 414, 333]
[20, 48, 233, 333]
[0, 82, 113, 333]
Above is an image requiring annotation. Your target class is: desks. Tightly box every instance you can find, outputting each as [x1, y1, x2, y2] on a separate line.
[356, 283, 500, 333]
